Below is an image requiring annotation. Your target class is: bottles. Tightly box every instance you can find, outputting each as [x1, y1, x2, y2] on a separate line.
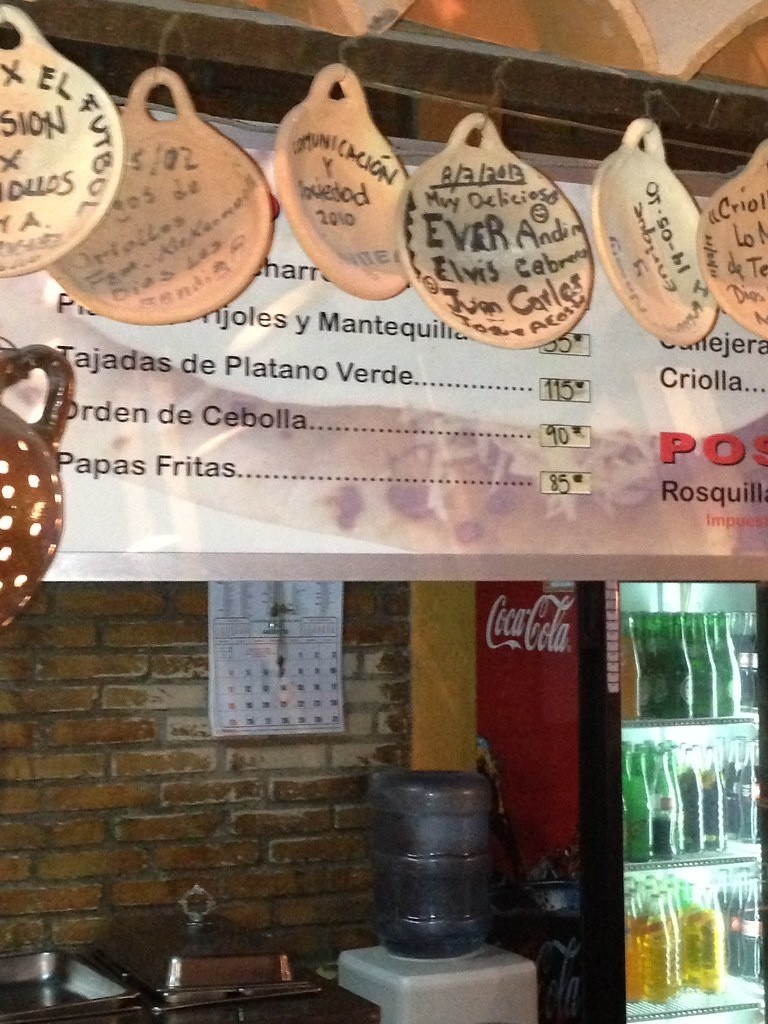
[617, 609, 766, 1003]
[370, 769, 498, 958]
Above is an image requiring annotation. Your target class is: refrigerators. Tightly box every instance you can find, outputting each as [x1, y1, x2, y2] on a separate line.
[472, 579, 768, 1022]
